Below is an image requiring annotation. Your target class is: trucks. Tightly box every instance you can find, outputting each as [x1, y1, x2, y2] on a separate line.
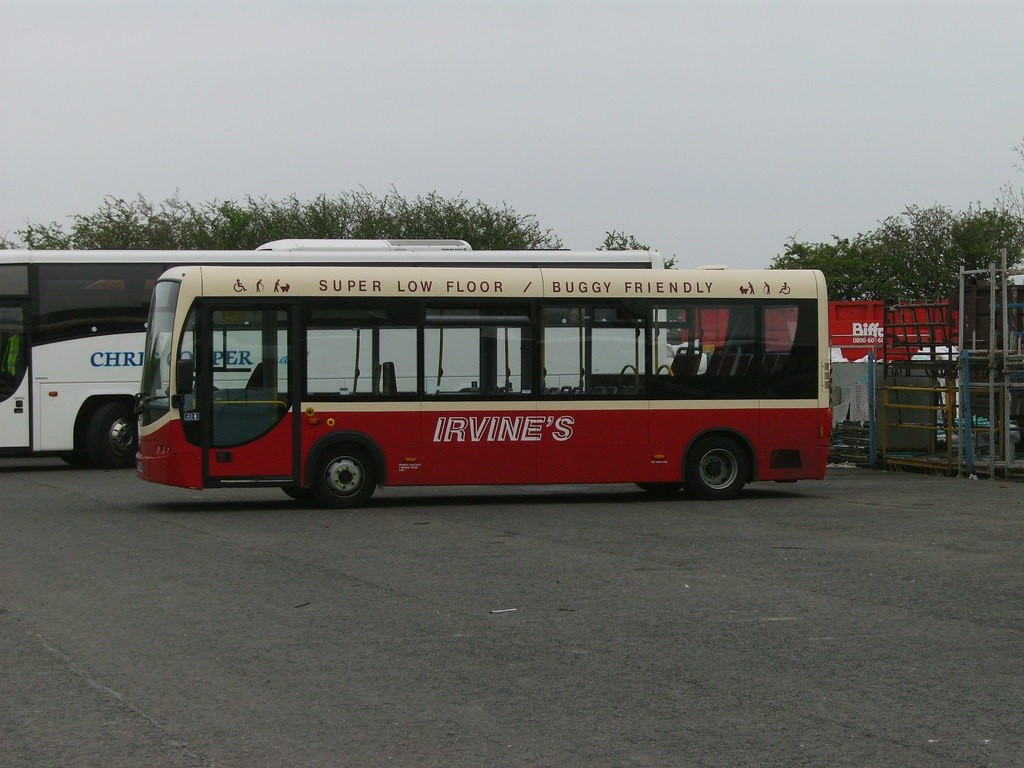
[680, 299, 968, 361]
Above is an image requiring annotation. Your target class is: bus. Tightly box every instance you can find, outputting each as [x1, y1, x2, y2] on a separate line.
[132, 263, 835, 508]
[0, 236, 668, 470]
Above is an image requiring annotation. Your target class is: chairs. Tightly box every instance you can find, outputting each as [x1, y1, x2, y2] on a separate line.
[460, 346, 780, 396]
[246, 363, 275, 401]
[383, 362, 398, 392]
